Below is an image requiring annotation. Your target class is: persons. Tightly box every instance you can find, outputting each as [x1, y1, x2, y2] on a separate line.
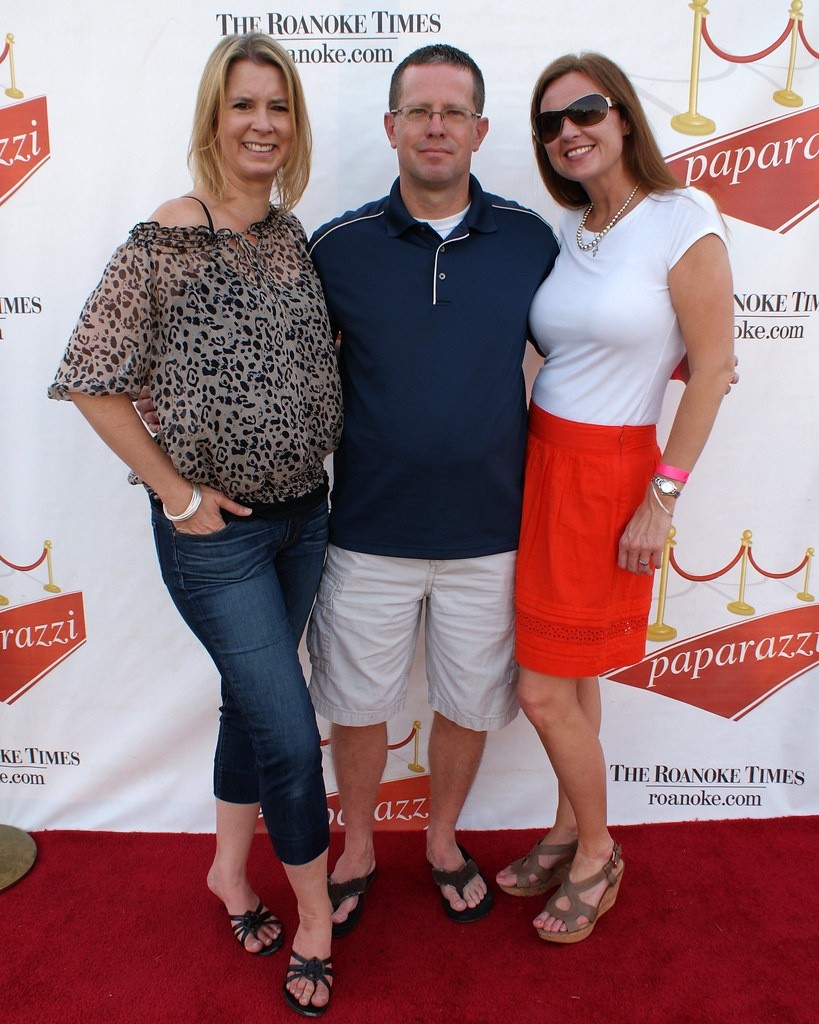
[495, 54, 733, 943]
[137, 43, 740, 936]
[49, 32, 341, 1018]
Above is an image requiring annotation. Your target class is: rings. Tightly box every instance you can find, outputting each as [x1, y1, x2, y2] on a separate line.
[639, 560, 649, 566]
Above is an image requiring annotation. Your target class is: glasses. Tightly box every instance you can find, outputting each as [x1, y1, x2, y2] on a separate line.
[391, 106, 480, 128]
[532, 93, 620, 143]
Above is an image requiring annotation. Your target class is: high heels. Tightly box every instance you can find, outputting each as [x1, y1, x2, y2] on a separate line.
[536, 838, 624, 942]
[497, 838, 581, 897]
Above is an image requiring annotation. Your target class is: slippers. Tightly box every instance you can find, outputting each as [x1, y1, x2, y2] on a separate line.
[432, 844, 493, 923]
[282, 949, 332, 1017]
[326, 859, 377, 935]
[229, 900, 283, 956]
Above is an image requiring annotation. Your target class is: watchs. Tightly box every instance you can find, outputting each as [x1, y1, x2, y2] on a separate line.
[651, 475, 680, 498]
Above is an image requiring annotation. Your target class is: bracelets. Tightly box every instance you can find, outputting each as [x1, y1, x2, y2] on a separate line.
[650, 482, 673, 516]
[673, 364, 680, 379]
[162, 482, 201, 522]
[655, 464, 690, 482]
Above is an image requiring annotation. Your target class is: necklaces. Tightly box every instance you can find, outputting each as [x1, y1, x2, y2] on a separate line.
[576, 184, 640, 250]
